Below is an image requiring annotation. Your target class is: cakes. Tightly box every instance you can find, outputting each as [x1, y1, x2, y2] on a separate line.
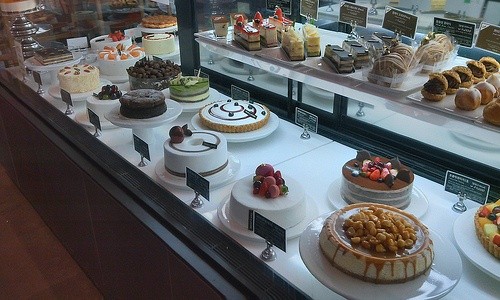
[57, 63, 100, 93]
[119, 89, 167, 119]
[319, 202, 434, 284]
[199, 99, 270, 133]
[142, 14, 177, 28]
[0, 0, 36, 12]
[341, 149, 414, 210]
[86, 84, 128, 122]
[88, 30, 146, 76]
[169, 67, 210, 102]
[142, 33, 175, 54]
[229, 163, 307, 231]
[126, 56, 183, 92]
[33, 46, 74, 66]
[474, 199, 500, 260]
[163, 124, 228, 177]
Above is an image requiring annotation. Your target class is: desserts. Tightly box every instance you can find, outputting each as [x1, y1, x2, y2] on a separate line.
[212, 7, 500, 126]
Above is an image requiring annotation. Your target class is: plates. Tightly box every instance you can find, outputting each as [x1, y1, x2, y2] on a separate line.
[155, 150, 242, 190]
[23, 24, 179, 102]
[326, 178, 429, 219]
[406, 90, 486, 121]
[452, 131, 500, 150]
[104, 99, 183, 128]
[194, 20, 472, 99]
[75, 107, 120, 130]
[191, 111, 280, 143]
[160, 88, 220, 112]
[299, 209, 462, 300]
[216, 193, 319, 242]
[454, 207, 500, 282]
[474, 117, 500, 133]
